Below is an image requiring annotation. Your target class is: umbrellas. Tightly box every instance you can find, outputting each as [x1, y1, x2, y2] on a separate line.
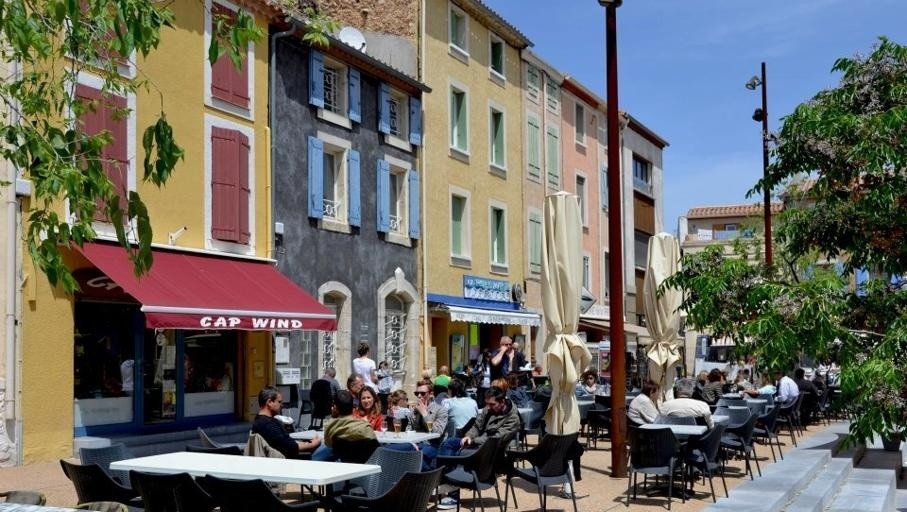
[541, 191, 593, 493]
[642, 232, 689, 407]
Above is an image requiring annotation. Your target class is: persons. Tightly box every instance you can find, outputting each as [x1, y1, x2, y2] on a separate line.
[217, 358, 235, 391]
[256, 335, 599, 506]
[120, 359, 140, 395]
[628, 355, 841, 475]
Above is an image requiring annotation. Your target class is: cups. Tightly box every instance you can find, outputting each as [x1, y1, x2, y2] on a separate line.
[323, 418, 329, 432]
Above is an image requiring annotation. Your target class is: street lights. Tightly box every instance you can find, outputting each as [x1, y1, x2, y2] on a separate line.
[741, 56, 774, 306]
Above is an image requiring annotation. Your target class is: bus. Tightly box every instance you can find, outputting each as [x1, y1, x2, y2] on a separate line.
[691, 330, 840, 389]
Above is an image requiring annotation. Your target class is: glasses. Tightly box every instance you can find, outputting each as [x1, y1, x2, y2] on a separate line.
[414, 391, 431, 398]
[503, 343, 513, 347]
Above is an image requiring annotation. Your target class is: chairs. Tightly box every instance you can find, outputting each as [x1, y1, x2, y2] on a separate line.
[0, 489, 46, 506]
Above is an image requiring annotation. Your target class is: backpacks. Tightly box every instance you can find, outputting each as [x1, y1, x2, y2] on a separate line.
[471, 347, 492, 377]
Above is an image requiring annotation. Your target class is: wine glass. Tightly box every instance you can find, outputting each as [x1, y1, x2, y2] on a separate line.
[380, 419, 435, 439]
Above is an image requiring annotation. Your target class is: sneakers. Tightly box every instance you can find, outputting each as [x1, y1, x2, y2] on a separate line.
[437, 497, 461, 511]
